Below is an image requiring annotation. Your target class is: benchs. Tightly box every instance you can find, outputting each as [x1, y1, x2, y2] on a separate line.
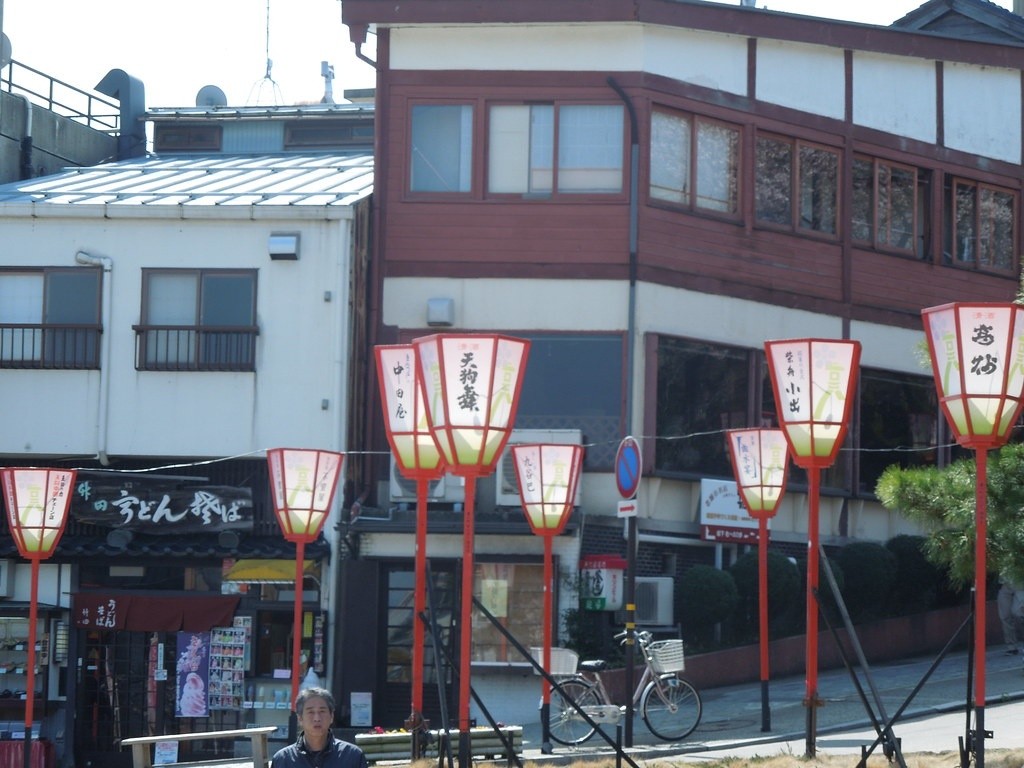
[355, 725, 523, 764]
[121, 725, 278, 768]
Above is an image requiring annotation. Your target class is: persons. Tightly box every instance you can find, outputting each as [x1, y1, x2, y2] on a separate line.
[210, 632, 242, 706]
[271, 688, 368, 768]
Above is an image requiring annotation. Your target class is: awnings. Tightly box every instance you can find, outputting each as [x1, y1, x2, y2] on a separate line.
[225, 559, 318, 585]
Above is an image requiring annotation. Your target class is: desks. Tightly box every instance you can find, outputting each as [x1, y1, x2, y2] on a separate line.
[0, 738, 55, 768]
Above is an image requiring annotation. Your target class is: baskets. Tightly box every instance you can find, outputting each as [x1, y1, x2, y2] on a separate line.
[645, 639, 685, 672]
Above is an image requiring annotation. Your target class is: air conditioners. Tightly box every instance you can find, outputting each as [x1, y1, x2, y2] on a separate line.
[496, 428, 584, 508]
[388, 446, 466, 504]
[613, 575, 675, 624]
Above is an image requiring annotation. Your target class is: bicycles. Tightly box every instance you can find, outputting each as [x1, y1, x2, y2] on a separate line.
[537, 628, 704, 746]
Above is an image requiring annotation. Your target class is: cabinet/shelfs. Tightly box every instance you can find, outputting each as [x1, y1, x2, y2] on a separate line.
[0, 619, 48, 698]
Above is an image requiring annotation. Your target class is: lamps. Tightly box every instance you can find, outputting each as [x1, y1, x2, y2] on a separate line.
[55, 621, 69, 663]
[427, 298, 454, 328]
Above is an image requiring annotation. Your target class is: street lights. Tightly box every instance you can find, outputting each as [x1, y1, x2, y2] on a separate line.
[764, 338, 864, 760]
[412, 333, 532, 768]
[920, 302, 1024, 768]
[0, 467, 74, 768]
[726, 426, 791, 735]
[372, 342, 446, 764]
[266, 446, 345, 735]
[511, 443, 586, 755]
